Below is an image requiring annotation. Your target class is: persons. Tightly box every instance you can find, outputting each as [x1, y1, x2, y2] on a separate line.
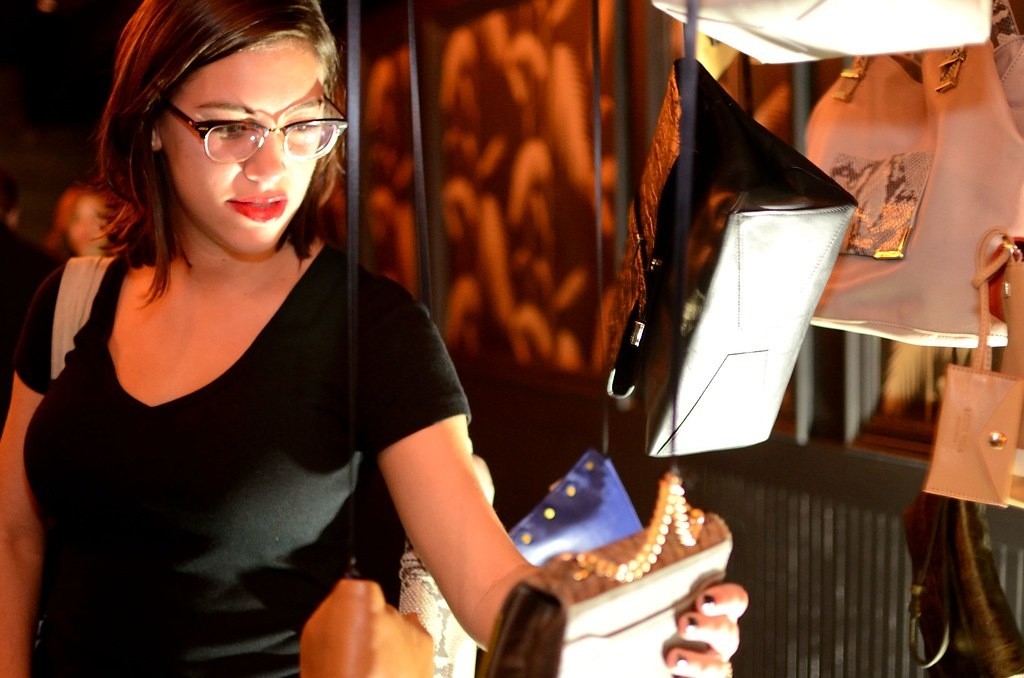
[0, 0, 748, 678]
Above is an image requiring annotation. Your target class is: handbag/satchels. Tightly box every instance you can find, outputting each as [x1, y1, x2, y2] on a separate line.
[593, 58, 859, 458]
[484, 473, 734, 678]
[921, 227, 1024, 510]
[300, 455, 495, 678]
[805, 0, 1024, 348]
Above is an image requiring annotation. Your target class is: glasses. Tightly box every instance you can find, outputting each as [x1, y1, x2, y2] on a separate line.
[161, 95, 348, 164]
